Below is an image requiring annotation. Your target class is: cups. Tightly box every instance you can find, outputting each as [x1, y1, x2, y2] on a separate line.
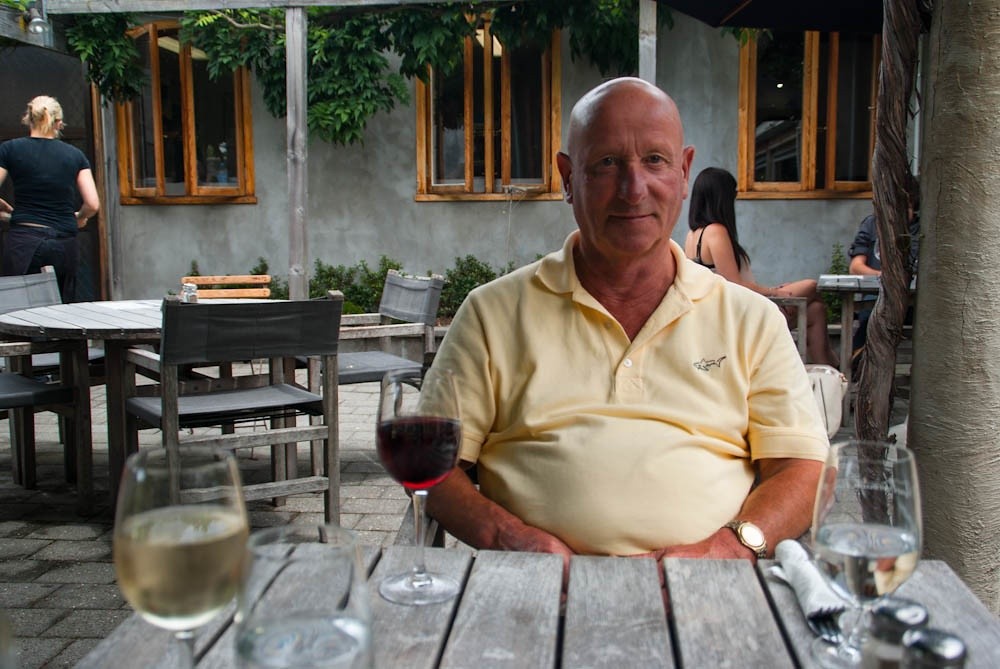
[234, 525, 374, 669]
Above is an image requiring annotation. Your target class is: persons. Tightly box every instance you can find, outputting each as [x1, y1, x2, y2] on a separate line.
[414, 78, 838, 559]
[0, 93, 102, 358]
[687, 164, 834, 375]
[839, 176, 930, 380]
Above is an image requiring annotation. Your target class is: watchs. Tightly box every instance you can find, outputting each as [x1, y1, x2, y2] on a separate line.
[720, 519, 770, 559]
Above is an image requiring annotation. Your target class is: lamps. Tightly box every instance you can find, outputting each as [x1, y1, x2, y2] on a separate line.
[23, 8, 49, 35]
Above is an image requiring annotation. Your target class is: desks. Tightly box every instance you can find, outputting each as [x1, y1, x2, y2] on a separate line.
[817, 274, 916, 426]
[72, 546, 1000, 669]
[0, 300, 307, 525]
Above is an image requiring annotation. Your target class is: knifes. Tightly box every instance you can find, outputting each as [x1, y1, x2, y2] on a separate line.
[807, 616, 845, 645]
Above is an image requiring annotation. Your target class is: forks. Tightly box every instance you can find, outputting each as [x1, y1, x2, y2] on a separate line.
[815, 607, 845, 619]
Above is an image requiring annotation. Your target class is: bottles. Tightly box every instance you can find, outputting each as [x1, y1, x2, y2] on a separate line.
[858, 597, 928, 669]
[900, 627, 967, 669]
[182, 283, 197, 302]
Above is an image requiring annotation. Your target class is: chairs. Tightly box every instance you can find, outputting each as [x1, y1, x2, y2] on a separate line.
[0, 266, 105, 444]
[124, 296, 344, 528]
[0, 373, 76, 515]
[181, 272, 271, 298]
[295, 270, 445, 462]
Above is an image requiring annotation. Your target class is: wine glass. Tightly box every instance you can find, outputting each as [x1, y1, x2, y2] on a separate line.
[376, 366, 464, 606]
[113, 444, 256, 669]
[810, 439, 923, 669]
